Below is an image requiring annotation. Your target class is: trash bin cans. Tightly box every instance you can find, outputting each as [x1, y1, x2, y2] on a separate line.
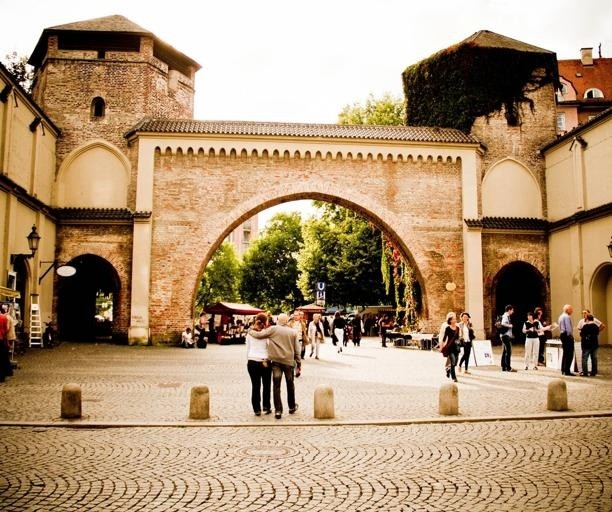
[545, 339, 575, 372]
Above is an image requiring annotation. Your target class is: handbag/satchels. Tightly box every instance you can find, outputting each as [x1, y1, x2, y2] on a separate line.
[494, 319, 508, 334]
[443, 344, 449, 357]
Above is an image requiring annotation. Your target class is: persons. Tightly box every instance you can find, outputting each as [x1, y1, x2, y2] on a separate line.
[286, 309, 417, 378]
[181, 310, 278, 349]
[245, 315, 272, 417]
[0, 303, 13, 382]
[1, 304, 16, 350]
[438, 304, 607, 383]
[248, 312, 302, 419]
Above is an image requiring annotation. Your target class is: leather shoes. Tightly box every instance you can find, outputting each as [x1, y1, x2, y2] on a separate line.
[562, 371, 597, 376]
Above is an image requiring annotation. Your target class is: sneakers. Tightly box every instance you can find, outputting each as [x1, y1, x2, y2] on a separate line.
[446, 366, 471, 382]
[510, 368, 517, 372]
[256, 404, 298, 418]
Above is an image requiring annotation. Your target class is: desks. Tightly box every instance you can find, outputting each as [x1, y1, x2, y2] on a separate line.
[386, 332, 432, 350]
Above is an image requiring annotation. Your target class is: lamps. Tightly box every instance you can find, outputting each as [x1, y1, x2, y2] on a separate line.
[607, 235, 612, 258]
[11, 225, 41, 262]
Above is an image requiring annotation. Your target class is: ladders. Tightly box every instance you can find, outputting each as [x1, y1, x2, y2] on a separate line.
[29, 293, 44, 348]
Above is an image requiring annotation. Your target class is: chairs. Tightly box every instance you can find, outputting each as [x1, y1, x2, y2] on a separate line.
[432, 335, 439, 348]
